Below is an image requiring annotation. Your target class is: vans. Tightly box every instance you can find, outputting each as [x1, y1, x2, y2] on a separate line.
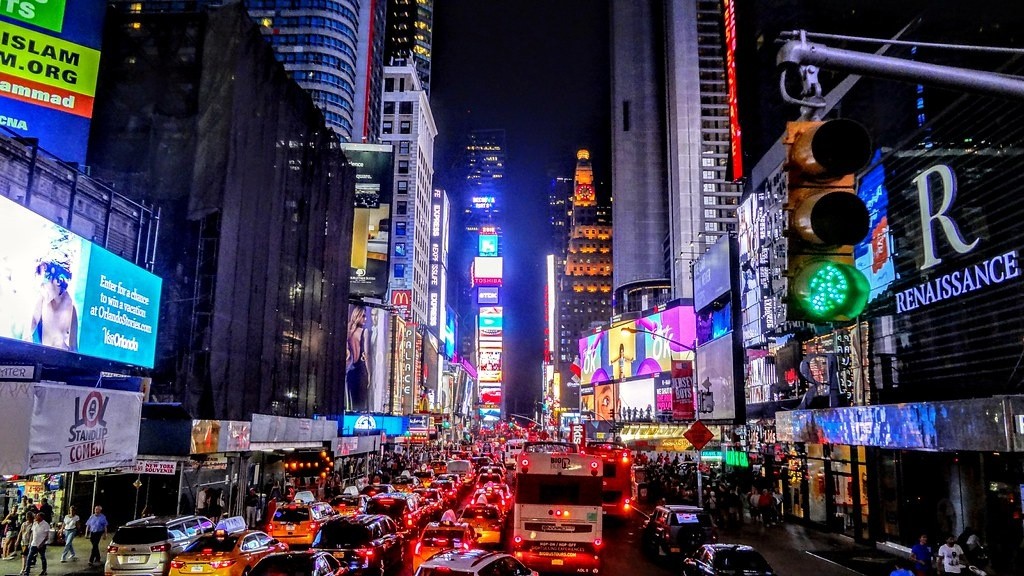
[312, 514, 409, 576]
[266, 491, 338, 547]
[104, 515, 218, 576]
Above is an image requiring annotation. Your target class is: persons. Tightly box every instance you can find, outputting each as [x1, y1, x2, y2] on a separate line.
[60, 506, 82, 562]
[612, 344, 633, 378]
[13, 247, 78, 350]
[346, 304, 379, 411]
[198, 487, 225, 522]
[633, 452, 785, 527]
[85, 506, 108, 567]
[909, 521, 989, 576]
[1, 496, 52, 576]
[264, 439, 467, 513]
[245, 487, 259, 529]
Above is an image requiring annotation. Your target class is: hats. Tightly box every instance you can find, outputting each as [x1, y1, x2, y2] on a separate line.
[250, 488, 254, 491]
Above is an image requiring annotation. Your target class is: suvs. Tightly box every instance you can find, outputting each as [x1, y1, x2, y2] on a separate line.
[640, 504, 720, 566]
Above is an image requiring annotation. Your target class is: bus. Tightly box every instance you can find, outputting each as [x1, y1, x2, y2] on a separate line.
[579, 439, 633, 526]
[511, 439, 605, 576]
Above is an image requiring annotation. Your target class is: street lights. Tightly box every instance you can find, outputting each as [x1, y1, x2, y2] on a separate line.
[581, 409, 620, 444]
[621, 327, 704, 508]
[509, 413, 545, 432]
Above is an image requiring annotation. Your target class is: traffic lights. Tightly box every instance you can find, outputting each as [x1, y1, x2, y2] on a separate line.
[780, 114, 874, 330]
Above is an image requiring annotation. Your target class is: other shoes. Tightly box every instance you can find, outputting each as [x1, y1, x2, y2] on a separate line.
[24, 572, 29, 575]
[61, 554, 66, 561]
[94, 557, 101, 562]
[88, 561, 93, 564]
[68, 552, 76, 559]
[20, 569, 24, 574]
[10, 551, 17, 556]
[3, 556, 14, 560]
[39, 571, 47, 575]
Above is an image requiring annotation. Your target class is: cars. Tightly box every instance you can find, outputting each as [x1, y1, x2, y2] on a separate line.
[413, 546, 539, 576]
[455, 492, 511, 555]
[330, 485, 372, 520]
[677, 542, 777, 576]
[167, 516, 290, 576]
[357, 438, 523, 537]
[412, 507, 481, 573]
[242, 549, 350, 576]
